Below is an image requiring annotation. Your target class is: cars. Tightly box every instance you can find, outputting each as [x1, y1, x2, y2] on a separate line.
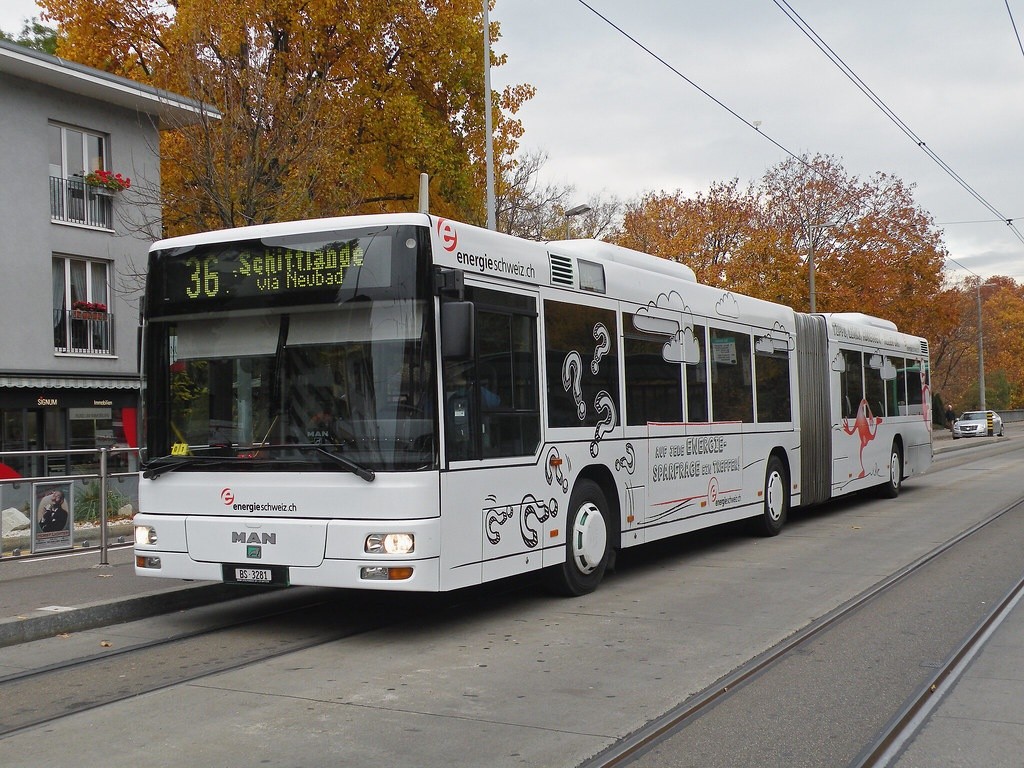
[952, 410, 1004, 438]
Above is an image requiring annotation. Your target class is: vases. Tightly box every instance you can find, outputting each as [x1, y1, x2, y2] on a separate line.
[90, 185, 116, 198]
[72, 309, 107, 320]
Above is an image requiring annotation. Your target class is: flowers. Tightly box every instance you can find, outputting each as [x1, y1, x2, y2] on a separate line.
[73, 300, 108, 313]
[84, 169, 130, 191]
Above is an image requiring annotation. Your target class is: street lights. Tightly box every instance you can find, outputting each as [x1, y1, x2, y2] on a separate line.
[807, 221, 836, 312]
[565, 203, 593, 240]
[976, 282, 998, 412]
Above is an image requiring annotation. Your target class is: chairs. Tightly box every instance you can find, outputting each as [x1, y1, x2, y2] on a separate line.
[840, 394, 885, 418]
[966, 417, 973, 420]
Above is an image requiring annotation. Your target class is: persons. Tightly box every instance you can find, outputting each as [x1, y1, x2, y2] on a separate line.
[415, 349, 501, 470]
[39, 490, 68, 532]
[945, 404, 955, 432]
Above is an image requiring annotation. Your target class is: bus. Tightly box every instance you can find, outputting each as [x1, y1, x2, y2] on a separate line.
[131, 210, 934, 600]
